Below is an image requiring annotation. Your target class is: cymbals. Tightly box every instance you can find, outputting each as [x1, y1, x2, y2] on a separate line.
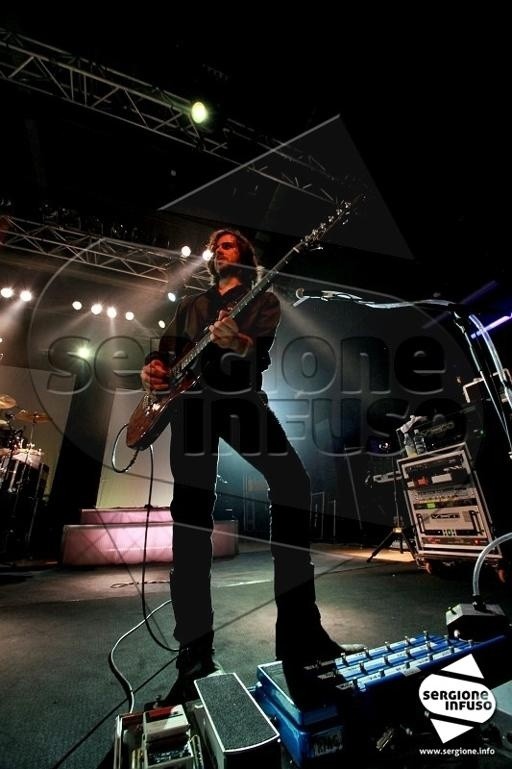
[0, 393, 16, 409]
[16, 411, 50, 424]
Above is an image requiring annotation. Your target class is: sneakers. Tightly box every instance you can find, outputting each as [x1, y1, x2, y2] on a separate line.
[276, 638, 368, 662]
[177, 654, 224, 691]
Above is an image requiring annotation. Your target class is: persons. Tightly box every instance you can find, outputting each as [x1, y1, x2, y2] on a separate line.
[141, 227, 346, 709]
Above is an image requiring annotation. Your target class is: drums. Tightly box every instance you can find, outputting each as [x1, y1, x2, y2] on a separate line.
[0, 455, 39, 501]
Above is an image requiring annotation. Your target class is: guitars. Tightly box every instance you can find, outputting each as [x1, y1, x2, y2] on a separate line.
[126, 193, 366, 451]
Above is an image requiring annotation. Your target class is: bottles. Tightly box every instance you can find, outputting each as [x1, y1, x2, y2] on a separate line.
[403, 429, 426, 458]
